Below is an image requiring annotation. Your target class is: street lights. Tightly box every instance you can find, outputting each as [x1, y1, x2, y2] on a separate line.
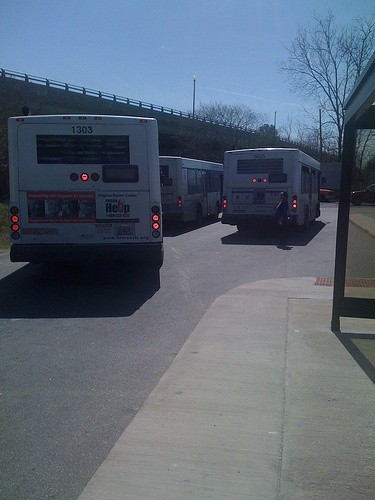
[191, 73, 197, 121]
[274, 108, 277, 129]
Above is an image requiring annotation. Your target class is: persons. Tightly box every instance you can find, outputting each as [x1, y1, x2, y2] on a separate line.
[272, 191, 288, 245]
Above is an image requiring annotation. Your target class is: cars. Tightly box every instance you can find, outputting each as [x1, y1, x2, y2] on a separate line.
[350, 184, 375, 205]
[320, 188, 335, 202]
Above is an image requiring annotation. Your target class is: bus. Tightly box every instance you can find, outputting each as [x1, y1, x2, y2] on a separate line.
[158, 155, 223, 225]
[6, 113, 164, 267]
[220, 146, 327, 232]
